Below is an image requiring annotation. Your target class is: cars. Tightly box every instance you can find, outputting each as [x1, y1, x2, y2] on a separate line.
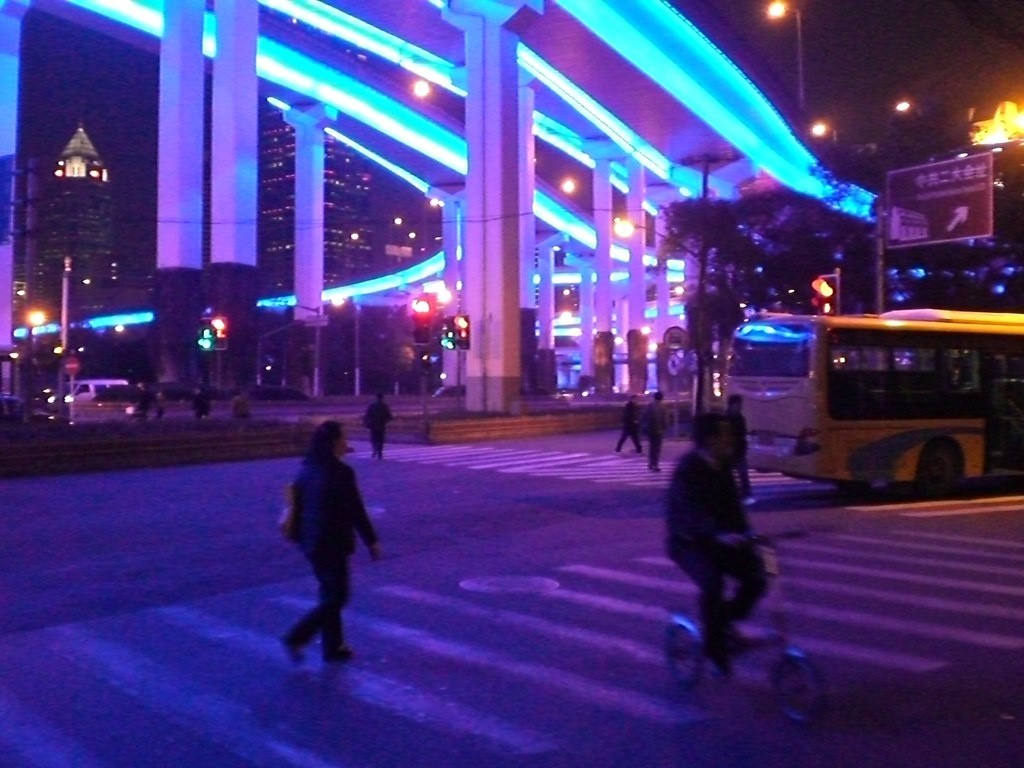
[431, 385, 466, 397]
[247, 384, 309, 401]
[93, 384, 165, 418]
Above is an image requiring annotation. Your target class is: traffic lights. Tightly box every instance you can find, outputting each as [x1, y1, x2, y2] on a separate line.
[210, 317, 228, 350]
[412, 298, 433, 344]
[199, 327, 214, 351]
[440, 315, 455, 350]
[453, 315, 471, 349]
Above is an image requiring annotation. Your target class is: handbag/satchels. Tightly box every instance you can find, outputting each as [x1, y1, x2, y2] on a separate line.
[277, 506, 299, 538]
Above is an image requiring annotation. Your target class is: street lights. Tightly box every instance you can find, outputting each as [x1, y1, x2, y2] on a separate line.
[613, 218, 707, 417]
[765, 1, 805, 109]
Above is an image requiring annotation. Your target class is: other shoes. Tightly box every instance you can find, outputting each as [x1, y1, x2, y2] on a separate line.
[742, 496, 755, 505]
[324, 646, 353, 658]
[648, 465, 661, 472]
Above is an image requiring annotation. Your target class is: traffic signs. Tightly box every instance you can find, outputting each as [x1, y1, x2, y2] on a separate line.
[884, 150, 993, 248]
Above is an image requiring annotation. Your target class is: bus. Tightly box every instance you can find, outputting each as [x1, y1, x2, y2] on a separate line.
[724, 306, 1024, 501]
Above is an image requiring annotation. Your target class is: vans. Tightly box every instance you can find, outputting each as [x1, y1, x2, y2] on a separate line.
[62, 378, 129, 401]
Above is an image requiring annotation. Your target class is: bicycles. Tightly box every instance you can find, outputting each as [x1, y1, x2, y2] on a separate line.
[662, 528, 826, 729]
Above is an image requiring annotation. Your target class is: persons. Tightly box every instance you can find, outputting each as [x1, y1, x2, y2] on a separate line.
[663, 411, 769, 681]
[191, 386, 213, 419]
[279, 419, 382, 664]
[644, 392, 665, 471]
[232, 391, 250, 418]
[615, 394, 643, 453]
[725, 393, 755, 497]
[363, 393, 392, 460]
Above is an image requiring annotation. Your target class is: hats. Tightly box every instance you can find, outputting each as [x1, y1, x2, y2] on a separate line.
[653, 391, 663, 400]
